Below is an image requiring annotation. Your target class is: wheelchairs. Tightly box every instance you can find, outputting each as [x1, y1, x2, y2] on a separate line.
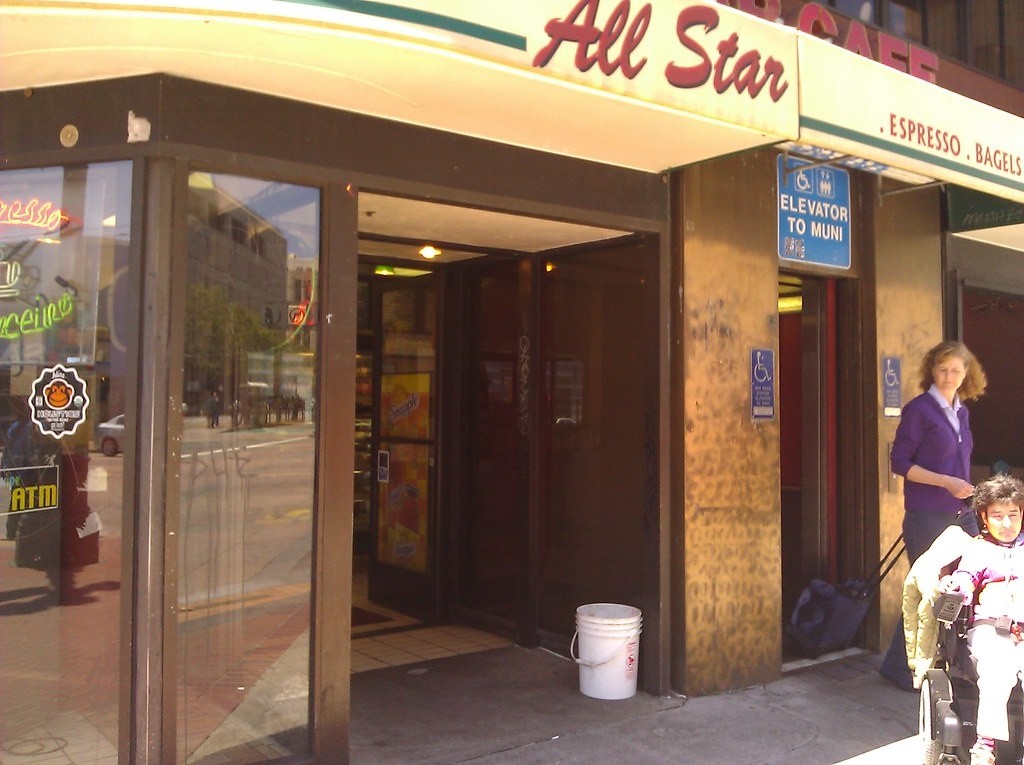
[917, 593, 1024, 765]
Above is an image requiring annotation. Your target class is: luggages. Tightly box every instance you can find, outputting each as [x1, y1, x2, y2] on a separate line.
[787, 532, 906, 660]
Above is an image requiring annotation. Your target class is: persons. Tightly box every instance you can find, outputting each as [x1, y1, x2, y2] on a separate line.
[937, 473, 1023, 765]
[204, 387, 221, 430]
[0, 398, 35, 538]
[879, 340, 987, 690]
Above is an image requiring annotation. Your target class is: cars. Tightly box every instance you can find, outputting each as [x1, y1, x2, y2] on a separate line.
[93, 414, 124, 456]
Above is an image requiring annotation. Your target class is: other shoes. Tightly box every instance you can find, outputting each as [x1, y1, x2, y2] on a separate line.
[878, 668, 921, 692]
[969, 743, 996, 765]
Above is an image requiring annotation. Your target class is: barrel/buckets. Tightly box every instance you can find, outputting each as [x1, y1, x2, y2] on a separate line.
[570, 601, 643, 701]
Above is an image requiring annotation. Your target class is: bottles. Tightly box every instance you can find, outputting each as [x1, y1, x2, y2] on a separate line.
[934, 588, 964, 628]
